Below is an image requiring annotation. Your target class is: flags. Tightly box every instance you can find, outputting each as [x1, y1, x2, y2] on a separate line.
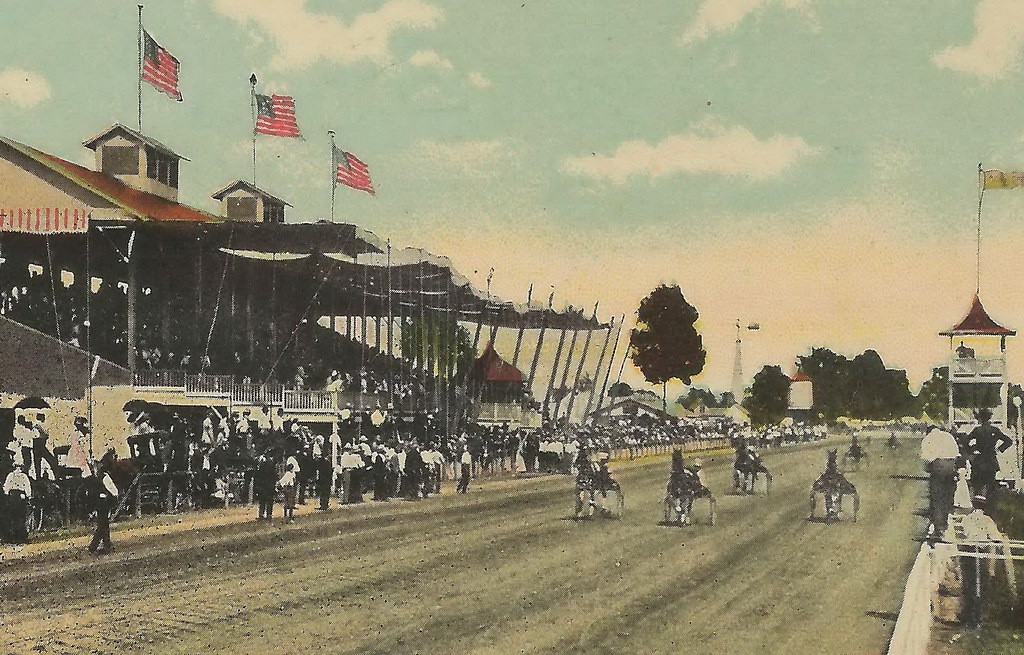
[333, 146, 375, 195]
[983, 170, 1024, 189]
[254, 94, 306, 141]
[141, 29, 183, 103]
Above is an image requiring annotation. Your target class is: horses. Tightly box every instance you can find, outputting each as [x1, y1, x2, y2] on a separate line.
[570, 432, 903, 525]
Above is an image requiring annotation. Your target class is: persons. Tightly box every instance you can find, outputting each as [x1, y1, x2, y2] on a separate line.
[921, 419, 962, 540]
[957, 495, 1003, 627]
[0, 253, 827, 554]
[964, 407, 1014, 496]
[955, 341, 966, 358]
